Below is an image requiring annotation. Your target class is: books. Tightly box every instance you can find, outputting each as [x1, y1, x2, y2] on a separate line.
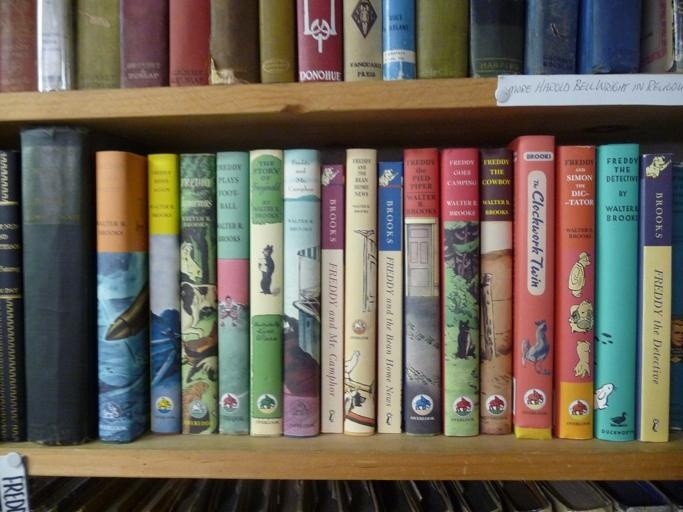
[28, 476, 682, 512]
[0, 127, 682, 446]
[2, 1, 683, 92]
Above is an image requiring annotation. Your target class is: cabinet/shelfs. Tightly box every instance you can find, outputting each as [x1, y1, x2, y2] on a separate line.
[0, 72, 683, 483]
